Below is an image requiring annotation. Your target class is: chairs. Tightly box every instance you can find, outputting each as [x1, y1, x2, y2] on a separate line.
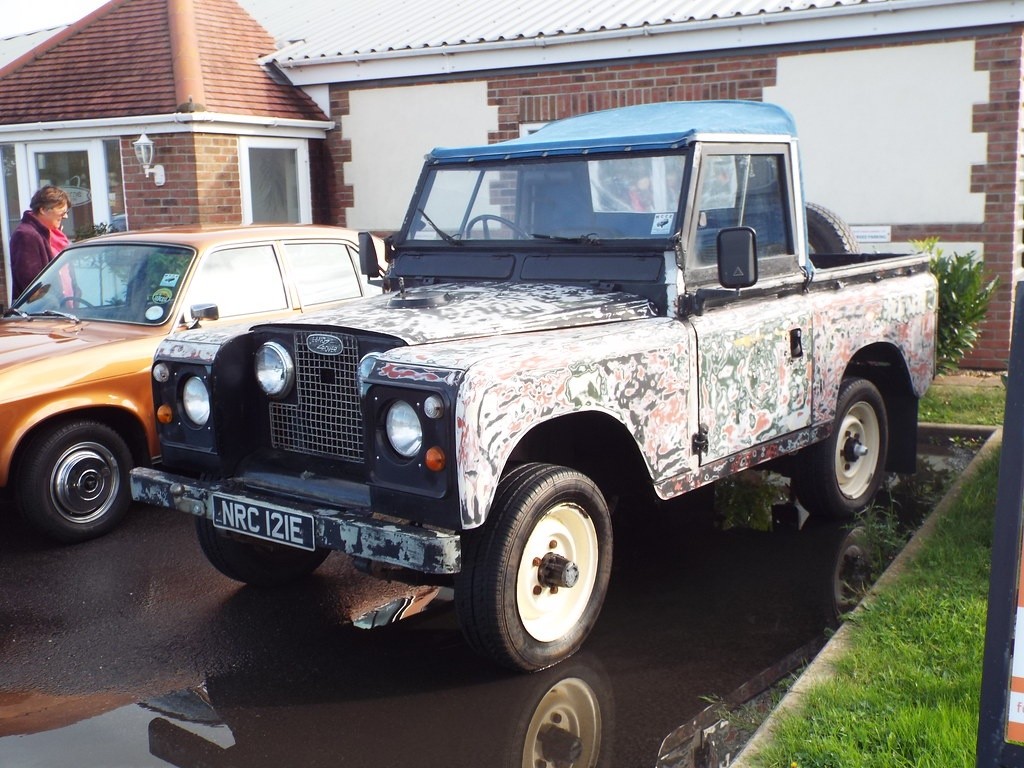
[520, 173, 774, 269]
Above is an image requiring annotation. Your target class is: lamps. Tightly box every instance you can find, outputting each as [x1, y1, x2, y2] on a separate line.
[133, 133, 166, 187]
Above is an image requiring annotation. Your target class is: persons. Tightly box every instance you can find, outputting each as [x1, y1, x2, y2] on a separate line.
[9, 184, 82, 309]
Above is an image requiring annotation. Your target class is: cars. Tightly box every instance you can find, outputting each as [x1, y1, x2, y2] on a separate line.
[0, 222, 397, 539]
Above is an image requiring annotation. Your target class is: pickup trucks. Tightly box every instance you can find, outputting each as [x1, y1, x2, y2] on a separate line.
[128, 101, 940, 677]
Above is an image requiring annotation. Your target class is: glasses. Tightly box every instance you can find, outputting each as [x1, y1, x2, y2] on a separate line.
[45, 209, 69, 216]
